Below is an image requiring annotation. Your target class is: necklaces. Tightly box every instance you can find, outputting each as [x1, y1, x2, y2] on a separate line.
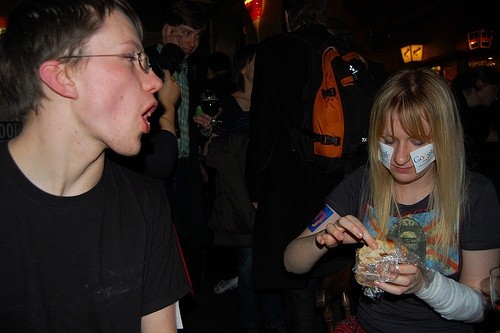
[391, 177, 437, 242]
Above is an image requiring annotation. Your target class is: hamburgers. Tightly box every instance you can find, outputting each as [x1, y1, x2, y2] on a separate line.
[354, 240, 410, 291]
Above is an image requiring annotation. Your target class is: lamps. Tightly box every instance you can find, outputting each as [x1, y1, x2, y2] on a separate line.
[400, 45, 424, 63]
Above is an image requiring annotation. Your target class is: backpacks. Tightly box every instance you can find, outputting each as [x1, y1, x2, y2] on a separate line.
[286, 32, 379, 181]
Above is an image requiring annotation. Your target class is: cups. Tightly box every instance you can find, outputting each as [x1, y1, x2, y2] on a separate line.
[489, 266, 500, 313]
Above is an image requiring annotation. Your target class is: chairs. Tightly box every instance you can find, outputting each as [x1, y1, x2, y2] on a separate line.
[314, 260, 369, 333]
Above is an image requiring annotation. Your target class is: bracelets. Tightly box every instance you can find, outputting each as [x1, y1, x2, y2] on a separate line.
[159, 117, 177, 132]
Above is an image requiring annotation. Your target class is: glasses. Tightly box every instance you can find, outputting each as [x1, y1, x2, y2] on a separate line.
[56, 51, 151, 72]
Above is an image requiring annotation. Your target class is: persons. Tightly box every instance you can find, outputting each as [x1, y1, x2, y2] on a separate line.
[194, 21, 292, 294]
[106, 43, 187, 182]
[282, 67, 500, 333]
[0, 0, 193, 333]
[454, 67, 500, 202]
[146, 11, 223, 275]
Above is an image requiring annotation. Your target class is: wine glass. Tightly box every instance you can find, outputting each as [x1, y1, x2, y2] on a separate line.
[200, 89, 222, 126]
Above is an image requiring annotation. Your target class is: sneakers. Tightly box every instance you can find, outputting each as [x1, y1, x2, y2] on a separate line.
[213, 276, 239, 294]
